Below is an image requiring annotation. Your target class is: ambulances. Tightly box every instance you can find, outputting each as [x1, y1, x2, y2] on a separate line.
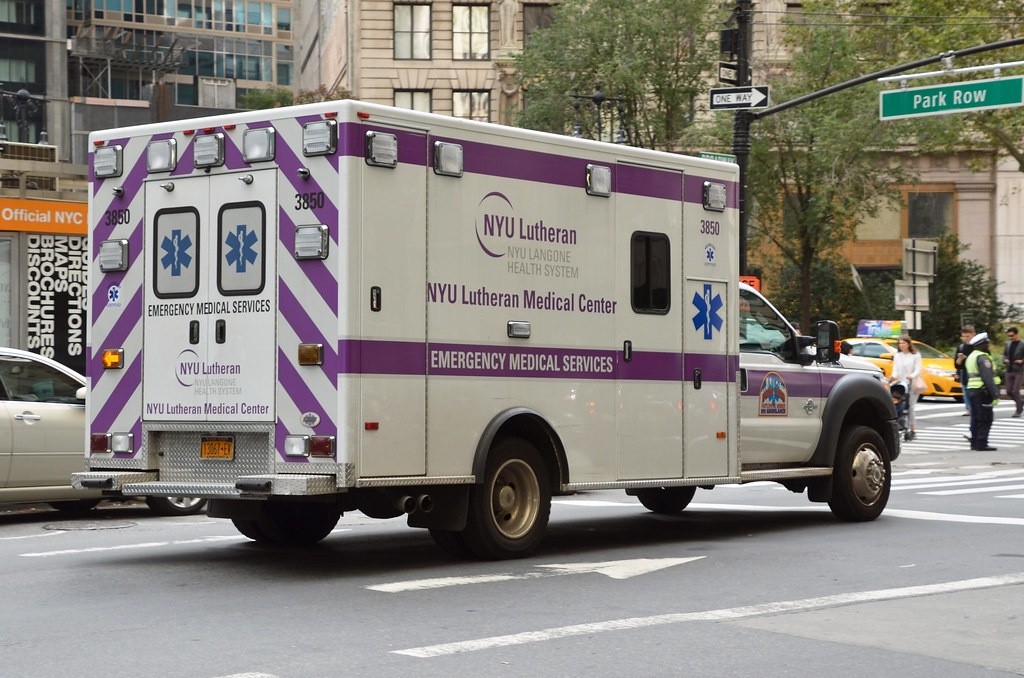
[70, 98, 900, 561]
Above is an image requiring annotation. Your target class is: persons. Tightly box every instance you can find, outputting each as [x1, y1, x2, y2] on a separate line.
[1002, 327, 1024, 417]
[889, 335, 922, 437]
[954, 325, 998, 451]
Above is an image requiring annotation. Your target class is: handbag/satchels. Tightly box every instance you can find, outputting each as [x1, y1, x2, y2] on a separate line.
[955, 344, 964, 382]
[912, 358, 928, 394]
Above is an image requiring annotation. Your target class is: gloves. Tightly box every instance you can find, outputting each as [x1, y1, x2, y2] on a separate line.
[992, 398, 999, 406]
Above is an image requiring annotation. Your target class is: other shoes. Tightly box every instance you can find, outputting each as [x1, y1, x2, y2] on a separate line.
[964, 431, 972, 439]
[1012, 409, 1024, 417]
[962, 410, 971, 415]
[977, 445, 996, 451]
[910, 430, 916, 437]
[971, 446, 976, 449]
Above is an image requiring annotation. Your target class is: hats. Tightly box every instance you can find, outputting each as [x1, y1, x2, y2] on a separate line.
[969, 332, 990, 346]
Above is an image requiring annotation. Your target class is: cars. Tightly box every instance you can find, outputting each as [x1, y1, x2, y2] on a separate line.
[841, 336, 964, 402]
[0, 347, 208, 517]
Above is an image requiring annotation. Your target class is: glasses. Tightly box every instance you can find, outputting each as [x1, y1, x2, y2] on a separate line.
[960, 336, 969, 340]
[1008, 335, 1015, 337]
